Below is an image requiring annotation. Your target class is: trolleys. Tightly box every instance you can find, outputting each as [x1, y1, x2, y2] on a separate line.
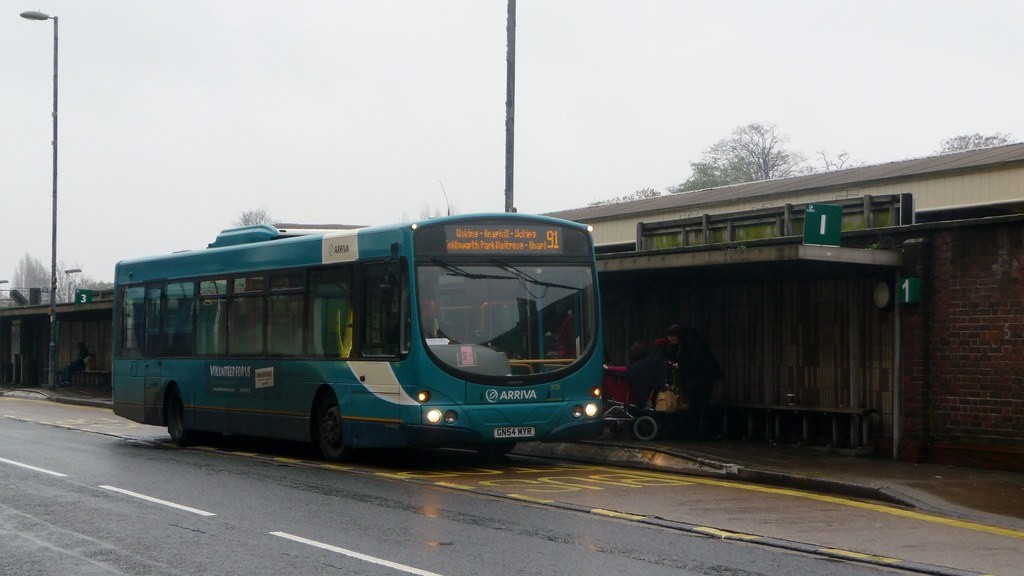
[602, 359, 685, 442]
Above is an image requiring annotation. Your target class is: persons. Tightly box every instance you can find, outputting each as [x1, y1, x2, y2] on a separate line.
[602, 343, 660, 411]
[422, 299, 440, 337]
[59, 342, 90, 387]
[666, 324, 724, 438]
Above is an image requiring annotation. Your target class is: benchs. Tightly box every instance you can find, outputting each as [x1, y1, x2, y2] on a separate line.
[714, 403, 876, 448]
[44, 368, 109, 387]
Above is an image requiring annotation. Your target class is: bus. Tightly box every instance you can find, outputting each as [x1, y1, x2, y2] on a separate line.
[112, 210, 606, 469]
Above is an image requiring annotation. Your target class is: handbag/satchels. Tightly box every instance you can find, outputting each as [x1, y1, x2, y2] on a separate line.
[655, 369, 681, 414]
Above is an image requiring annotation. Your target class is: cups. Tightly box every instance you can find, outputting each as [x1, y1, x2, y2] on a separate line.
[787, 394, 795, 406]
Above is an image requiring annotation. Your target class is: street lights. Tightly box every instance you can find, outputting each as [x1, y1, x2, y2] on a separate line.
[20, 10, 59, 394]
